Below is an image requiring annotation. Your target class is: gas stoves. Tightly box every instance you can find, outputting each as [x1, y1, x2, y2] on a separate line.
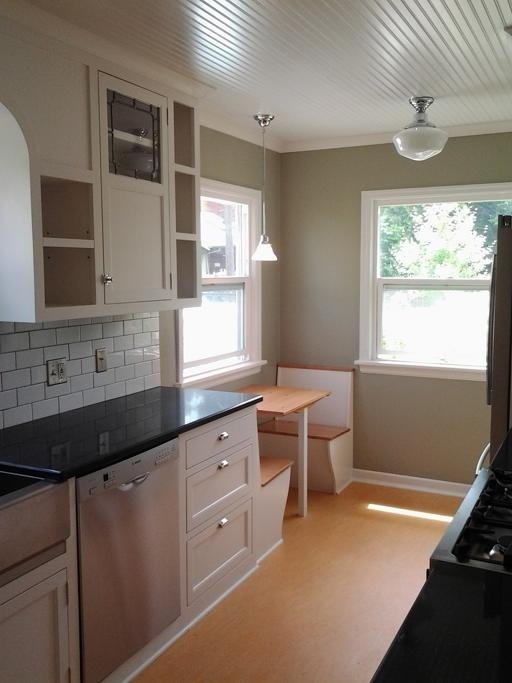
[452, 471, 512, 570]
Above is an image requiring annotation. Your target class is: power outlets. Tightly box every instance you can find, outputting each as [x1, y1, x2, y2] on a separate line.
[50, 440, 71, 465]
[47, 358, 67, 386]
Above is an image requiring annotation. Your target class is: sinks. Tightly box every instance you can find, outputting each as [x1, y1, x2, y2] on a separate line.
[1, 469, 51, 510]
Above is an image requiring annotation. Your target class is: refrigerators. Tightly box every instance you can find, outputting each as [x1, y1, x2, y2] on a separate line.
[75, 437, 180, 683]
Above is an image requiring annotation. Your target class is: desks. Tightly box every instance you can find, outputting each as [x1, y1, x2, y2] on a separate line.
[233, 385, 330, 518]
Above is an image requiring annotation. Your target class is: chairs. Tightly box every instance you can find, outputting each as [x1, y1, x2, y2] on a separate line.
[255, 456, 295, 563]
[257, 362, 357, 496]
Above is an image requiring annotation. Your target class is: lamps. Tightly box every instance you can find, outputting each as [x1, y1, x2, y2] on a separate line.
[251, 113, 277, 263]
[393, 96, 449, 163]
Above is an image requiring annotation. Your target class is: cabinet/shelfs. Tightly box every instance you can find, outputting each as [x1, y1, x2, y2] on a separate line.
[178, 405, 262, 637]
[0, 478, 71, 682]
[0, 33, 202, 323]
